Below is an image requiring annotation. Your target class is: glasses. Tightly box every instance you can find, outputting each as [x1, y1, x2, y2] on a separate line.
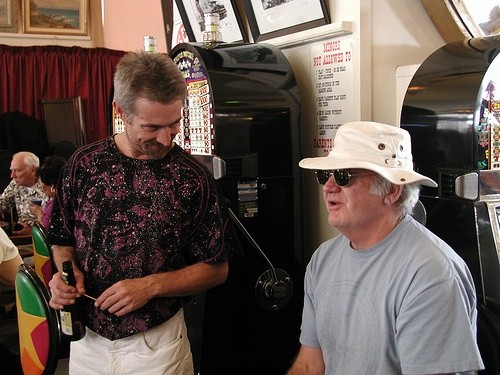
[314, 169, 377, 188]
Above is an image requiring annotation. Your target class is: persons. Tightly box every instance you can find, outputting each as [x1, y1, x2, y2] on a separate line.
[0, 227, 24, 286]
[0, 151, 53, 235]
[288, 122, 486, 375]
[29, 155, 68, 229]
[45, 50, 229, 375]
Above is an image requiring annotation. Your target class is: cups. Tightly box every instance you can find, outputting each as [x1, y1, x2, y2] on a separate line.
[31, 200, 42, 206]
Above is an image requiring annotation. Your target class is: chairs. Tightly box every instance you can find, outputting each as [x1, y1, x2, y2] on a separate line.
[11, 223, 62, 375]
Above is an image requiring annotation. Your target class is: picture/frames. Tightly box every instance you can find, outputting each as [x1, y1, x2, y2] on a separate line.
[22, 0, 90, 36]
[244, 0, 331, 42]
[175, 0, 249, 48]
[0, 0, 20, 37]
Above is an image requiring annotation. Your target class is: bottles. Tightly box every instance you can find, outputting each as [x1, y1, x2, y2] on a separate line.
[58, 260, 86, 341]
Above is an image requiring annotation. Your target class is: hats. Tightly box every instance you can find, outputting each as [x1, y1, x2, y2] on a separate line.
[298, 122, 438, 188]
